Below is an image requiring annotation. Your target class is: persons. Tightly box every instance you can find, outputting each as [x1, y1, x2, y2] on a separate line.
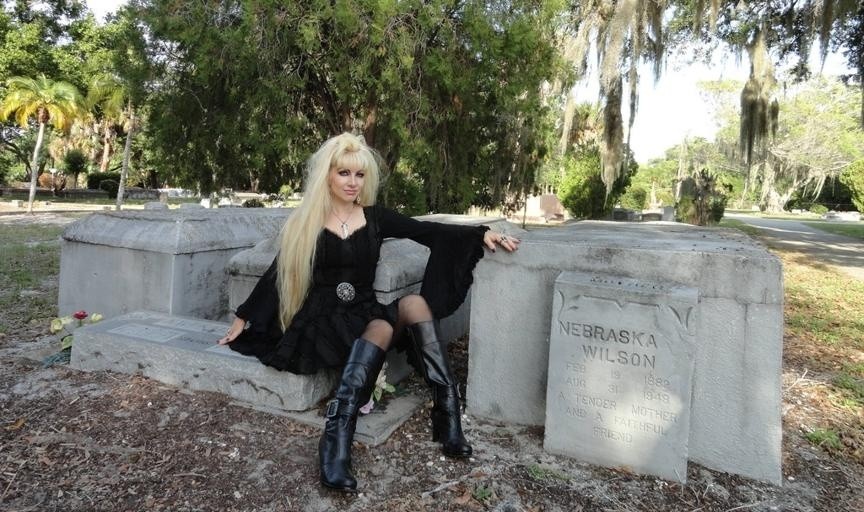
[216, 130, 522, 492]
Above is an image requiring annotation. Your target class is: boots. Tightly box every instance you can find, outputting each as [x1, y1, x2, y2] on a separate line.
[319, 338, 386, 493]
[406, 320, 473, 458]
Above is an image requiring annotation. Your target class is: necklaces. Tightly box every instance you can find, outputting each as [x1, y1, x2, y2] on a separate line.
[331, 207, 354, 239]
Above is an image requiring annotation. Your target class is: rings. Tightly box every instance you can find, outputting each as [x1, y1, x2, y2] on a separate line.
[499, 235, 507, 245]
[225, 327, 232, 336]
